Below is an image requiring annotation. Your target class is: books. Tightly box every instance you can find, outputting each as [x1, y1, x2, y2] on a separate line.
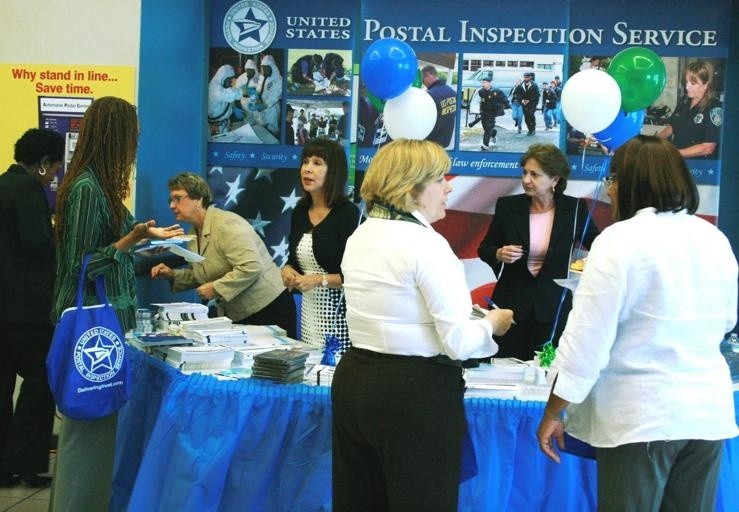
[127, 300, 337, 387]
[457, 355, 554, 401]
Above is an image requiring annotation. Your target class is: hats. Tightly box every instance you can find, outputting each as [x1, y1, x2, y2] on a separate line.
[286, 104, 296, 111]
[524, 73, 530, 76]
[483, 78, 490, 82]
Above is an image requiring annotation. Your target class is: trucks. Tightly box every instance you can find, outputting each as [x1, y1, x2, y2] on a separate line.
[461, 67, 551, 110]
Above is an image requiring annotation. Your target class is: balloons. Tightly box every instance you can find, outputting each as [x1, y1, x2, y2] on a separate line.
[560, 69, 621, 139]
[598, 109, 647, 153]
[360, 38, 419, 101]
[606, 46, 666, 112]
[382, 87, 436, 143]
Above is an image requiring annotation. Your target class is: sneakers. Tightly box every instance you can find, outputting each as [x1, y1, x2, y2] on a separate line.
[527, 130, 536, 136]
[518, 128, 522, 134]
[18, 472, 53, 486]
[0, 473, 21, 487]
[545, 121, 560, 131]
[515, 121, 518, 127]
[492, 130, 497, 144]
[480, 144, 489, 150]
[46, 253, 134, 420]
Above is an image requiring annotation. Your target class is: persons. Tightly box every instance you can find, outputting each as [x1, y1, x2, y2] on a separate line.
[332, 139, 514, 512]
[579, 58, 600, 71]
[208, 54, 282, 136]
[281, 138, 363, 356]
[567, 128, 615, 157]
[151, 173, 288, 325]
[286, 54, 350, 147]
[478, 143, 600, 361]
[479, 71, 563, 150]
[50, 97, 184, 510]
[538, 134, 739, 511]
[0, 128, 65, 487]
[659, 59, 724, 159]
[421, 66, 455, 148]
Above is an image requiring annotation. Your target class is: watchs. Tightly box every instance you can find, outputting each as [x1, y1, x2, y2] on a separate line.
[322, 276, 329, 289]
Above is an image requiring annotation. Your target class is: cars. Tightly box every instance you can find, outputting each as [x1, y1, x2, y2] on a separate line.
[290, 106, 343, 128]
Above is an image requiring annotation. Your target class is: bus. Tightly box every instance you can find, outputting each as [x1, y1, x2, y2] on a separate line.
[462, 53, 537, 81]
[535, 60, 556, 85]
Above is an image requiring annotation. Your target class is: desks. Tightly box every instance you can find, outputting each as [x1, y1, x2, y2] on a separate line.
[111, 334, 739, 511]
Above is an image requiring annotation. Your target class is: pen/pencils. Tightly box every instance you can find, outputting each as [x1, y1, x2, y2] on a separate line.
[484, 296, 517, 325]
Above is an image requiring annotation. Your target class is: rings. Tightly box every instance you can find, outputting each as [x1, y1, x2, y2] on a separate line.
[145, 223, 148, 228]
